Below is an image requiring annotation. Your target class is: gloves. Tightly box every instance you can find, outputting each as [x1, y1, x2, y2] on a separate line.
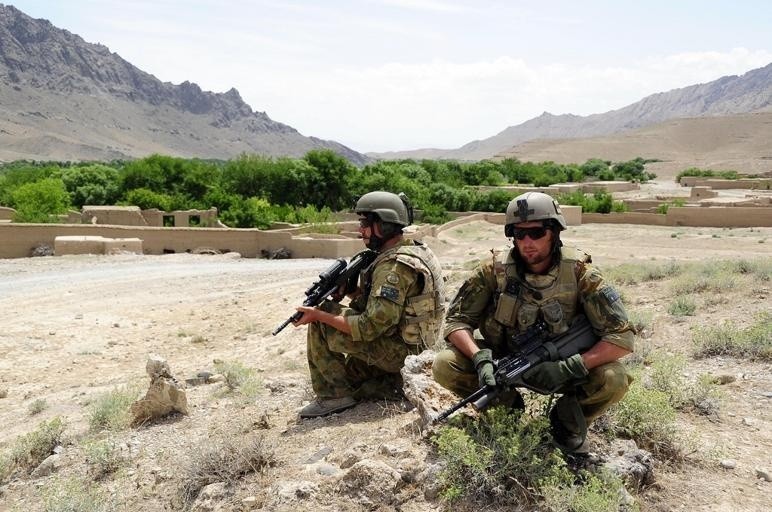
[522, 353, 590, 391]
[473, 349, 510, 404]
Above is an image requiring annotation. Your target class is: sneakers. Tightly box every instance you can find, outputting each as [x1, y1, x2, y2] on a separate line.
[301, 391, 356, 416]
[549, 404, 590, 453]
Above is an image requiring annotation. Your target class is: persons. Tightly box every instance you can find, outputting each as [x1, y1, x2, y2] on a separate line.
[292, 190, 445, 417]
[430, 191, 639, 457]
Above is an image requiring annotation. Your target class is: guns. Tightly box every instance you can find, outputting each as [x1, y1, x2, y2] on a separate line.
[432, 320, 601, 429]
[272, 250, 380, 336]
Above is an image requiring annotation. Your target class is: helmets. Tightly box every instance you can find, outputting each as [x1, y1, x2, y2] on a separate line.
[354, 190, 409, 227]
[505, 192, 568, 237]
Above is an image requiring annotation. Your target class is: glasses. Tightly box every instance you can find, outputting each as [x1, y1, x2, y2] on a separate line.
[512, 225, 549, 241]
